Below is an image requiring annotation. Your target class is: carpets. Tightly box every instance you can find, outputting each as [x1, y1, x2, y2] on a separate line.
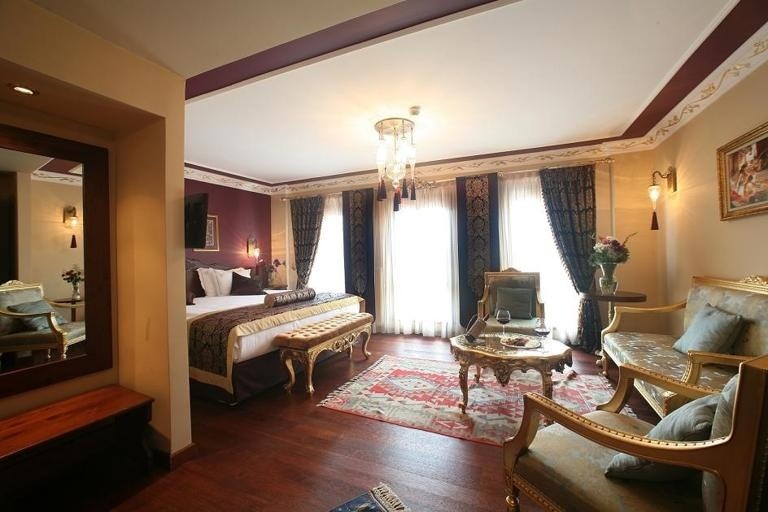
[318, 354, 639, 458]
[328, 483, 417, 511]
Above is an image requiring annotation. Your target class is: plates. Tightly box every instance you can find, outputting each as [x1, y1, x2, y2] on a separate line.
[499, 337, 540, 349]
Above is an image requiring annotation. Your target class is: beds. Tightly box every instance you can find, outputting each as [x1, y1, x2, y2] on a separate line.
[185, 259, 367, 408]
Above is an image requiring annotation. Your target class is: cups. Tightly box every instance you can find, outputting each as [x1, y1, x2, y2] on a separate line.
[496, 310, 510, 335]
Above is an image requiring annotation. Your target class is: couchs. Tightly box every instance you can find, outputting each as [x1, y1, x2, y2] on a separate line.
[596, 275, 768, 419]
[0, 280, 83, 358]
[478, 268, 545, 337]
[503, 354, 767, 512]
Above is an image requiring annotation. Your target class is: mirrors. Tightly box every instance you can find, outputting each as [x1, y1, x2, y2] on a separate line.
[1, 147, 88, 379]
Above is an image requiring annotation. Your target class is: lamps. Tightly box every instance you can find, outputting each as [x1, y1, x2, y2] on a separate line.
[495, 170, 504, 178]
[647, 166, 676, 231]
[254, 248, 266, 290]
[373, 118, 418, 212]
[62, 205, 81, 249]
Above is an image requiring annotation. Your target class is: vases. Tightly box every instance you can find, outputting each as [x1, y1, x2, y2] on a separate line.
[599, 262, 620, 296]
[70, 282, 83, 301]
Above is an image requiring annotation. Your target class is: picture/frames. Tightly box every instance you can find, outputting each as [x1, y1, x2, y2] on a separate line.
[193, 215, 220, 252]
[715, 121, 768, 223]
[247, 238, 258, 257]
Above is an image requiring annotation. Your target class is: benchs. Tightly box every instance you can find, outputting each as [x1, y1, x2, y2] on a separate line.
[273, 311, 373, 396]
[2, 384, 156, 500]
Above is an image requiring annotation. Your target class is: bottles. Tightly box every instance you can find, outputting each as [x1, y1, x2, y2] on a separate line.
[465, 313, 490, 343]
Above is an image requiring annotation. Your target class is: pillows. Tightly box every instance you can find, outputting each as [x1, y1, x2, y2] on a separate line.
[210, 267, 250, 297]
[265, 288, 315, 308]
[604, 393, 720, 480]
[494, 288, 533, 319]
[671, 302, 742, 354]
[8, 299, 67, 329]
[197, 266, 244, 297]
[231, 271, 267, 295]
[701, 372, 738, 511]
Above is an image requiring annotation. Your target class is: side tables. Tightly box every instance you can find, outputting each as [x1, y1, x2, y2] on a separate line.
[591, 289, 646, 330]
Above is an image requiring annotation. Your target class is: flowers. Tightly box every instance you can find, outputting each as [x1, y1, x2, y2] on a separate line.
[585, 231, 638, 278]
[60, 266, 86, 285]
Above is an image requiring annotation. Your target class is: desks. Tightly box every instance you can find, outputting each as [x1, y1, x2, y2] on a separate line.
[50, 296, 85, 322]
